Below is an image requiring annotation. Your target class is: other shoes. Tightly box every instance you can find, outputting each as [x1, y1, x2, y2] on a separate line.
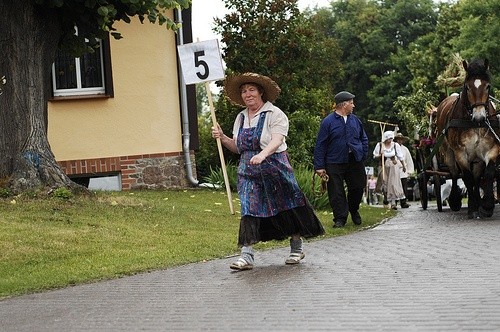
[350, 210, 362, 225]
[230, 253, 254, 269]
[285, 249, 305, 263]
[333, 220, 344, 228]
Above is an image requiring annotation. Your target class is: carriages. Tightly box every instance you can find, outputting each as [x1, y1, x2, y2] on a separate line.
[413, 57, 500, 219]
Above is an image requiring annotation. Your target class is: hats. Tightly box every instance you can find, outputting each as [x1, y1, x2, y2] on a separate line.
[382, 130, 394, 143]
[335, 91, 355, 101]
[224, 73, 282, 106]
[393, 133, 410, 143]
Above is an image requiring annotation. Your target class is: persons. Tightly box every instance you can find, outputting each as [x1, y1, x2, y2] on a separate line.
[314, 91, 369, 228]
[365, 130, 415, 210]
[211, 72, 326, 270]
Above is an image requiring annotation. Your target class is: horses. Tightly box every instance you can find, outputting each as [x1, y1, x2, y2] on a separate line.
[435, 58, 500, 219]
